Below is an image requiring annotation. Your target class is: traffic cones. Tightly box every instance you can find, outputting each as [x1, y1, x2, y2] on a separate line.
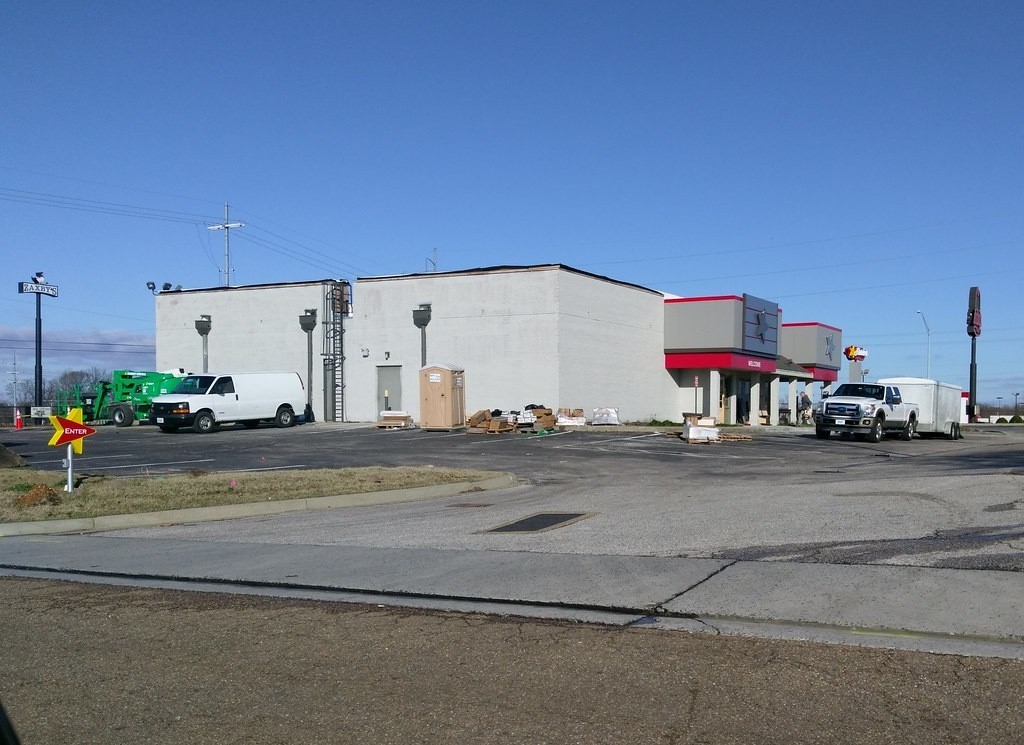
[15, 408, 24, 429]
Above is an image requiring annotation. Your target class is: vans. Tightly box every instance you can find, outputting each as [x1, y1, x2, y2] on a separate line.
[150, 371, 308, 435]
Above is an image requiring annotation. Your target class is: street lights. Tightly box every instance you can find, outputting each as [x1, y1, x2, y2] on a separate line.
[916, 310, 931, 379]
[1011, 393, 1020, 416]
[860, 368, 870, 383]
[996, 397, 1003, 419]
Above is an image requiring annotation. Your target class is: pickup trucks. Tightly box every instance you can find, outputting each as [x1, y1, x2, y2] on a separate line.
[814, 382, 920, 443]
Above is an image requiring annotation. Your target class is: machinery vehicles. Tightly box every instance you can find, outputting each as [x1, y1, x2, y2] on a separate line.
[56, 369, 198, 428]
